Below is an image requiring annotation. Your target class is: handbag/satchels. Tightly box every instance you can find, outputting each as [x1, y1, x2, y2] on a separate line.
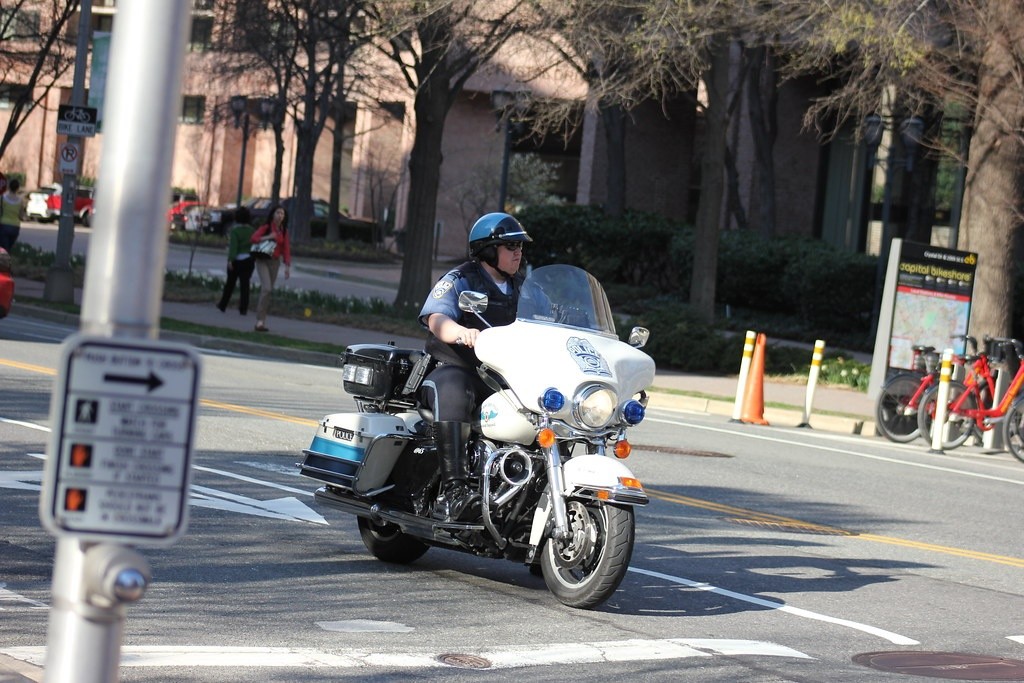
[250, 238, 278, 260]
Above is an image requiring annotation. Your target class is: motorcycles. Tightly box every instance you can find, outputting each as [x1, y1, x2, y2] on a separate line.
[295, 263, 655, 609]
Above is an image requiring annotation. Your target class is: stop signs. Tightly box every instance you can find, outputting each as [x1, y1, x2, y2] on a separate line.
[0, 173, 7, 194]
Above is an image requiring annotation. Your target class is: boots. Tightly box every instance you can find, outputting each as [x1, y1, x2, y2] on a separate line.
[433, 420, 484, 523]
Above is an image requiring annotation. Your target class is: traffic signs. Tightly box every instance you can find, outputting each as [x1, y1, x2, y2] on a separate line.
[40, 334, 202, 546]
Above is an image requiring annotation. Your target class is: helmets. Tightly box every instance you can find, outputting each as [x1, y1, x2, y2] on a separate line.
[469, 212, 534, 258]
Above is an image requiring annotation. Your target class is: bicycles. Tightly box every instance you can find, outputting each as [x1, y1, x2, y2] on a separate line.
[917, 335, 1024, 461]
[874, 333, 1021, 444]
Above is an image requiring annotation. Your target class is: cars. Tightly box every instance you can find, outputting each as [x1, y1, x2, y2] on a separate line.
[27, 185, 62, 222]
[185, 206, 208, 232]
[48, 185, 94, 226]
[170, 200, 207, 230]
[251, 196, 384, 246]
[202, 196, 285, 239]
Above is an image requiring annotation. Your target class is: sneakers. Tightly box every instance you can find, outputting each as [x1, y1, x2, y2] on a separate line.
[254, 324, 270, 332]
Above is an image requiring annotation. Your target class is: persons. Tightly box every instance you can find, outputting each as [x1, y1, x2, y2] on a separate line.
[417, 212, 553, 524]
[250, 205, 291, 332]
[0, 179, 24, 255]
[215, 206, 256, 315]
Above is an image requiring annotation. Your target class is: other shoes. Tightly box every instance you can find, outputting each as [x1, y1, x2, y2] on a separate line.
[215, 302, 227, 313]
[238, 306, 248, 315]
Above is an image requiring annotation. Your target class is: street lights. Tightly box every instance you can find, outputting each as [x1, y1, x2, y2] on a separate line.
[229, 95, 271, 209]
[863, 112, 923, 350]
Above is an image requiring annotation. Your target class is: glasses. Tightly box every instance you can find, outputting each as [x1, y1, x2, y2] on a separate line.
[501, 240, 524, 251]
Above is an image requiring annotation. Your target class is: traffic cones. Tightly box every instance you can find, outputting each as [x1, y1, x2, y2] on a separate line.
[729, 333, 769, 424]
